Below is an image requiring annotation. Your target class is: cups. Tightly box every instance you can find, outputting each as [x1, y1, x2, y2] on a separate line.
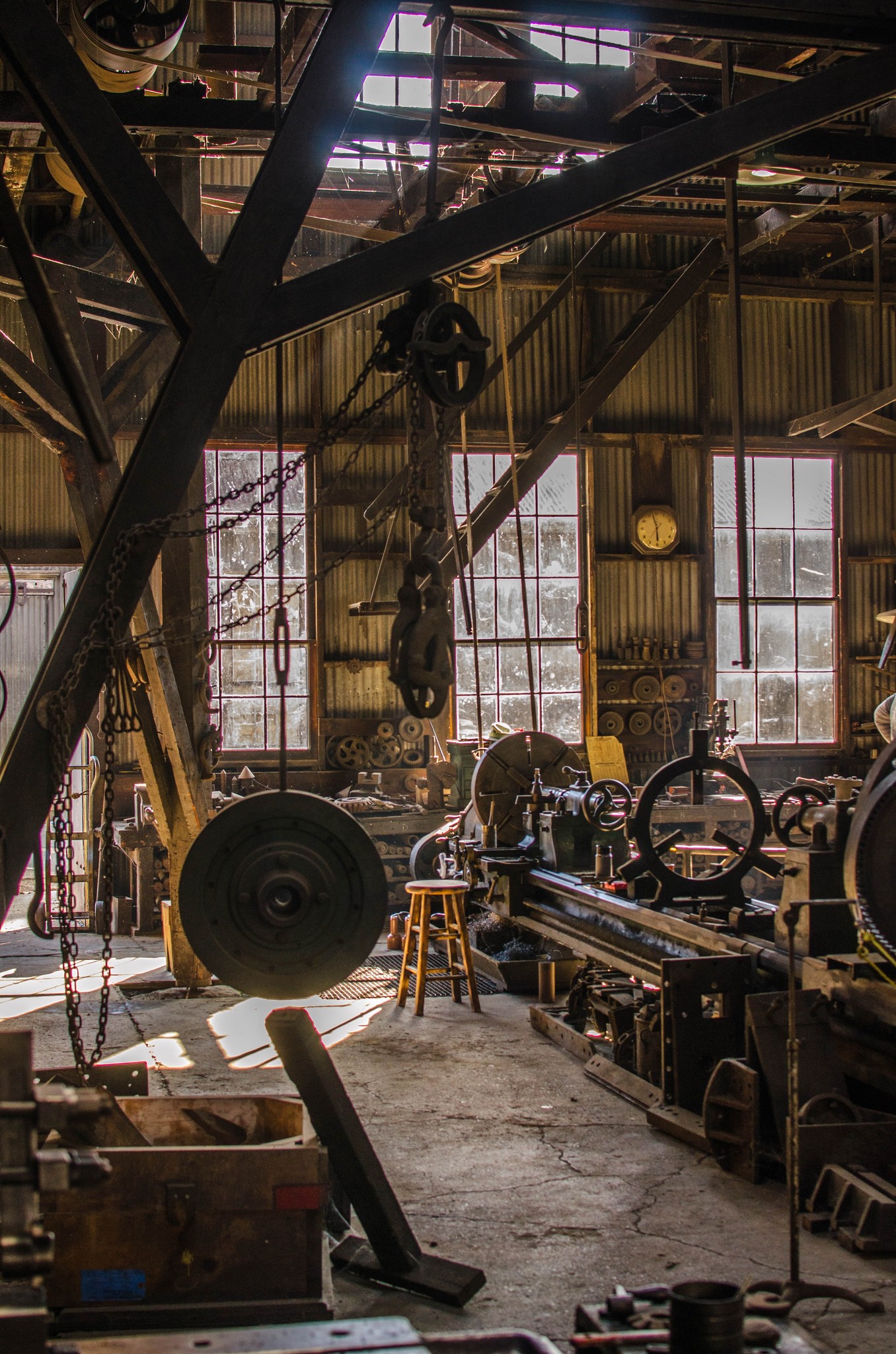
[595, 845, 612, 877]
[669, 1277, 744, 1354]
[482, 824, 497, 848]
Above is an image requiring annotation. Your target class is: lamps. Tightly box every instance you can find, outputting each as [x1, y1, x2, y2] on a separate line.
[737, 143, 806, 185]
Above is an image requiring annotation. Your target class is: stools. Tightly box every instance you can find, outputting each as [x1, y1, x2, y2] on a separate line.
[397, 880, 481, 1017]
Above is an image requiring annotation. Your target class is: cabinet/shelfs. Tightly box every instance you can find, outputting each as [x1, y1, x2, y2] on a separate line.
[112, 812, 456, 936]
[592, 552, 709, 669]
[844, 555, 896, 737]
[318, 488, 413, 669]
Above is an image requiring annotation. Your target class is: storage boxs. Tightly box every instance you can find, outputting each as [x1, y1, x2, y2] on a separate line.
[445, 740, 491, 808]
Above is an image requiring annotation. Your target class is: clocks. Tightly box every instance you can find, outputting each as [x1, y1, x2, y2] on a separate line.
[629, 504, 681, 555]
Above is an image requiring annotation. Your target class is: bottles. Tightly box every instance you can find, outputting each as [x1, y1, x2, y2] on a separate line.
[879, 636, 885, 654]
[867, 636, 875, 656]
